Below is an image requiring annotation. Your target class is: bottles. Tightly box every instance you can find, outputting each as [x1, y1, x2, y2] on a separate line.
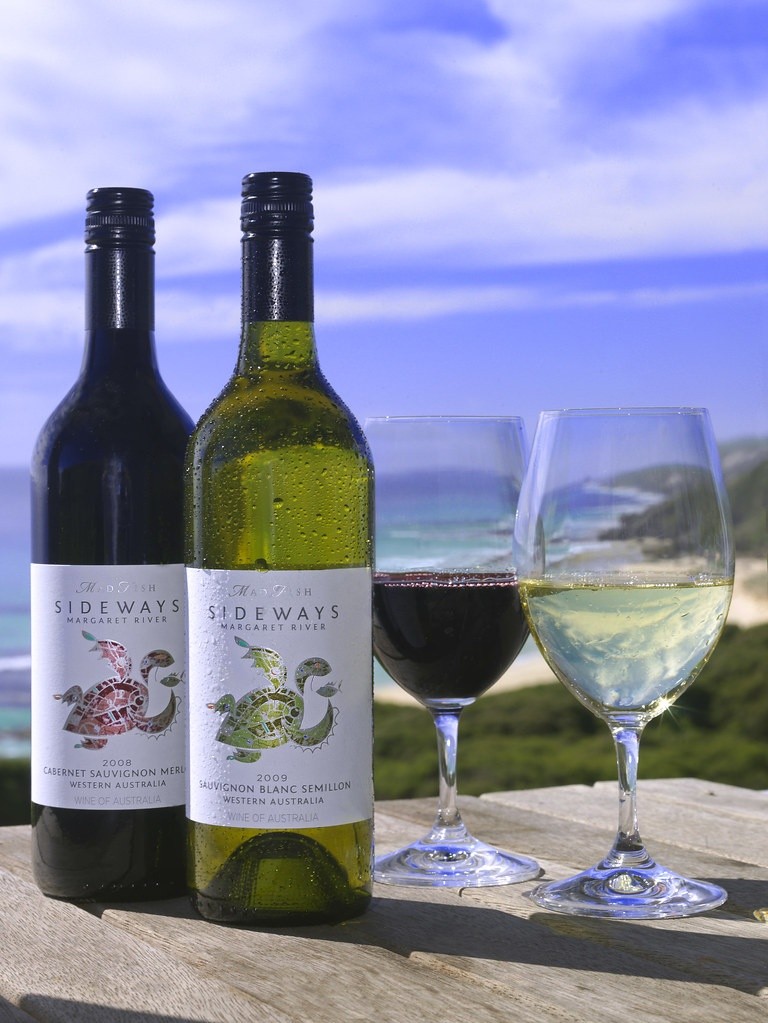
[28, 186, 199, 904]
[184, 173, 372, 926]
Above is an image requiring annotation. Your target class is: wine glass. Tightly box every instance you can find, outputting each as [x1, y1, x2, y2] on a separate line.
[351, 411, 554, 888]
[513, 404, 736, 920]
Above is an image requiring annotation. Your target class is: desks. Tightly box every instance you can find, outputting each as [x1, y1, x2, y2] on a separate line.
[0, 779, 768, 1023]
